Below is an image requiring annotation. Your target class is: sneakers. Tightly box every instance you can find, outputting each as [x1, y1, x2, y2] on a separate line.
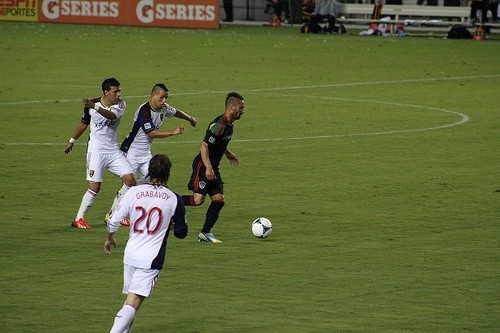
[120, 218, 130, 226]
[104, 213, 112, 225]
[198, 233, 222, 242]
[72, 218, 91, 228]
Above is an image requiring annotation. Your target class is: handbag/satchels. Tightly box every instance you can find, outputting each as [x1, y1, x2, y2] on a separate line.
[447, 24, 472, 40]
[327, 25, 345, 33]
[300, 24, 310, 33]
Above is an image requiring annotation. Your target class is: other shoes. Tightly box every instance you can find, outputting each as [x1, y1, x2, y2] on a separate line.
[222, 19, 233, 22]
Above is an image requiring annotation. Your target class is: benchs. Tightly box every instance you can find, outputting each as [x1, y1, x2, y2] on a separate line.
[317, 3, 500, 34]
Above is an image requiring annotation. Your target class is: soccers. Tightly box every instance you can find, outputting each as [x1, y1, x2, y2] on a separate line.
[250, 216, 273, 239]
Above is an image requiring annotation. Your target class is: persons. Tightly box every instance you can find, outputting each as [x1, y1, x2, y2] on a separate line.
[274, 0, 290, 24]
[104, 83, 198, 226]
[181, 92, 245, 243]
[291, 0, 303, 24]
[342, 0, 500, 39]
[64, 78, 137, 229]
[309, 0, 338, 35]
[222, 0, 234, 22]
[103, 154, 188, 333]
